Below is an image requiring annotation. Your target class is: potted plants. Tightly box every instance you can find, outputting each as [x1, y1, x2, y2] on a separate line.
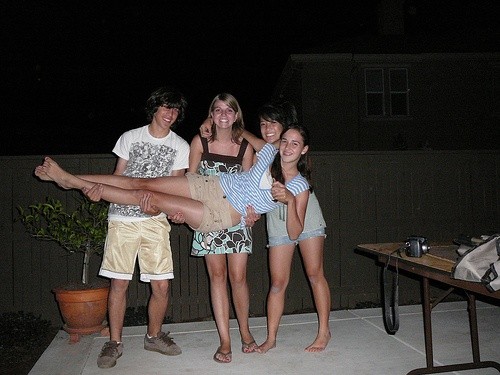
[14, 194, 110, 334]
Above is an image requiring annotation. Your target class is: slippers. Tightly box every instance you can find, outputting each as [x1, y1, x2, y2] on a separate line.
[213, 346, 231, 363]
[242, 337, 258, 353]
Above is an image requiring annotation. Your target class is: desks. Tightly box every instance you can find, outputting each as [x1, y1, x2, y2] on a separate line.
[354, 239, 500, 375]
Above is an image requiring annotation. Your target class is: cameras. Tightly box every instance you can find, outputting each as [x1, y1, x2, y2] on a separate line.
[405, 235, 432, 258]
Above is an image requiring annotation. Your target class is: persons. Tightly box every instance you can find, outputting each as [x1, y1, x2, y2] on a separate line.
[167, 92, 262, 363]
[251, 99, 331, 352]
[82, 88, 191, 369]
[34, 117, 312, 234]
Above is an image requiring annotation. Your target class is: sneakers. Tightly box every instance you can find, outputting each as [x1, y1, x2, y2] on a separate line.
[97, 340, 124, 369]
[144, 331, 181, 356]
